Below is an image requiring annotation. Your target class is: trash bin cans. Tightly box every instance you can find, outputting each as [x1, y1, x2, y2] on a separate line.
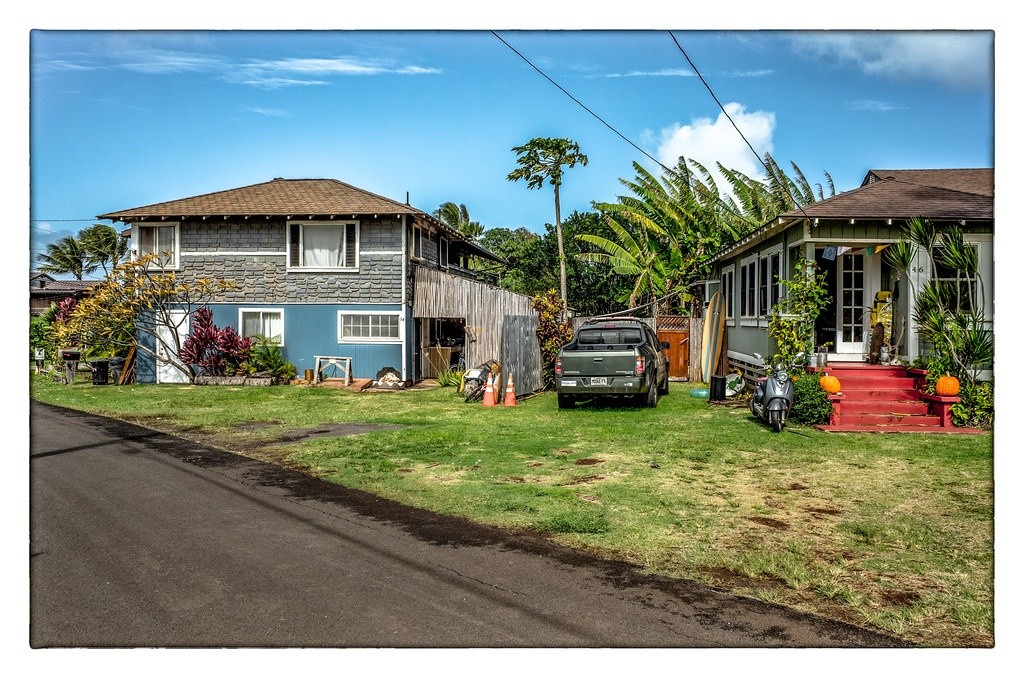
[89, 360, 109, 385]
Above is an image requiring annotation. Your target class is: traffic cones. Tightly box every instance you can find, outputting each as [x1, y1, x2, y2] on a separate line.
[505, 373, 516, 406]
[483, 372, 501, 406]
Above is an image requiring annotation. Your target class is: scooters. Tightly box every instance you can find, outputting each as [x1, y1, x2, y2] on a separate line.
[750, 352, 805, 432]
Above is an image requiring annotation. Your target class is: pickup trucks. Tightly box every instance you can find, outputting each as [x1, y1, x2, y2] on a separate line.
[555, 316, 670, 410]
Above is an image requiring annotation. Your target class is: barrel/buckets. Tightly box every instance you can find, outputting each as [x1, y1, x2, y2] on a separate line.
[817, 353, 827, 367]
[305, 369, 313, 380]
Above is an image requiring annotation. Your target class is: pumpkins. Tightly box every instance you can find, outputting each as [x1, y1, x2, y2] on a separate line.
[820, 372, 840, 393]
[936, 371, 959, 396]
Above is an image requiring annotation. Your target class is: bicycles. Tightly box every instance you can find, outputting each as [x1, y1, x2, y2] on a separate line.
[446, 340, 465, 375]
[465, 374, 487, 403]
